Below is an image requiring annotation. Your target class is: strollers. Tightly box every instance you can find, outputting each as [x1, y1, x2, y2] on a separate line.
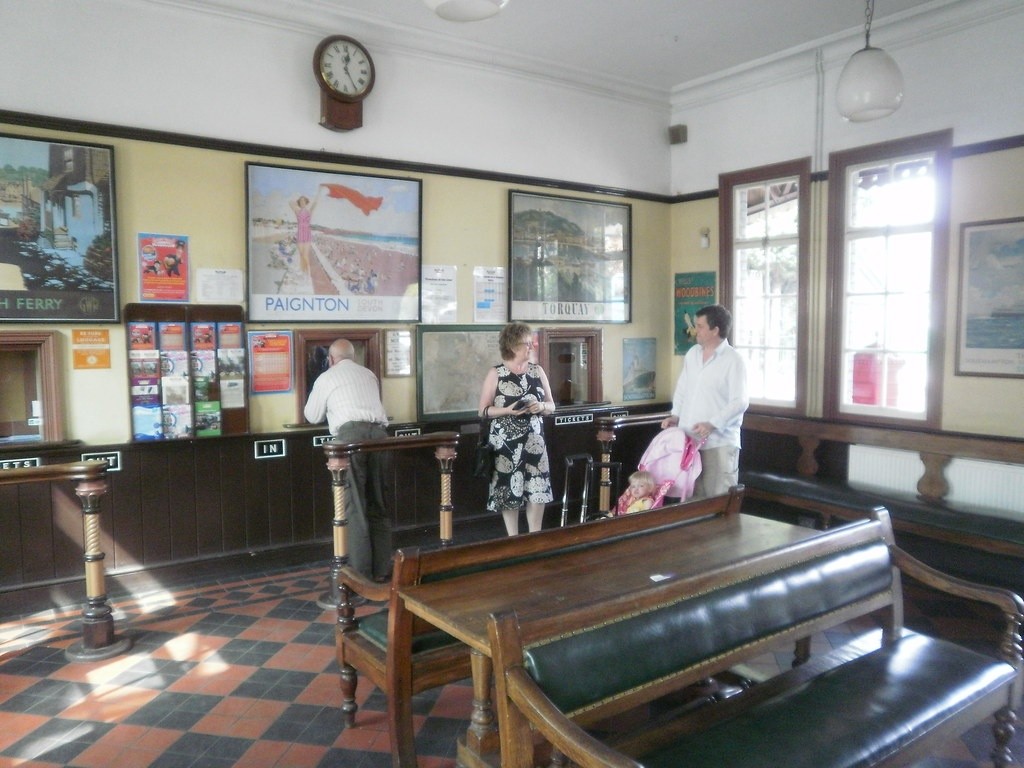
[575, 419, 713, 521]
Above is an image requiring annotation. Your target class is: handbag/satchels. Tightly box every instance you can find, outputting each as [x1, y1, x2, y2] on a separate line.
[474, 406, 494, 493]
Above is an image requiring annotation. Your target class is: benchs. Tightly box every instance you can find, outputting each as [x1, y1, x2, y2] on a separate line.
[336, 411, 1024, 768]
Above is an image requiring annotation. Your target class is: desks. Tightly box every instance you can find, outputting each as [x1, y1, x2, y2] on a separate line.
[396, 512, 828, 768]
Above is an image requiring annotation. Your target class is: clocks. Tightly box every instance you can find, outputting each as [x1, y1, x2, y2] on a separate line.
[312, 34, 376, 131]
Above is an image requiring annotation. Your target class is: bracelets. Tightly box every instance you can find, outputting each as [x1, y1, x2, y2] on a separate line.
[542, 402, 546, 411]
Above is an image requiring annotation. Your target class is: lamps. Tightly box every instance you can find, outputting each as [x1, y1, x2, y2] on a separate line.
[835, 0, 904, 122]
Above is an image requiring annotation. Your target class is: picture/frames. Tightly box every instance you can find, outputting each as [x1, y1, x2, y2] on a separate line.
[954, 216, 1024, 379]
[243, 161, 423, 323]
[416, 324, 505, 420]
[508, 189, 634, 325]
[0, 131, 122, 325]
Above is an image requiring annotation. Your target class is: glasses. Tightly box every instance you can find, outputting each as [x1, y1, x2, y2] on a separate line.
[515, 341, 534, 348]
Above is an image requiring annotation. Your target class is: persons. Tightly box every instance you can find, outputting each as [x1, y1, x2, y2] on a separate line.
[304, 338, 389, 583]
[477, 322, 556, 537]
[626, 471, 655, 513]
[661, 306, 750, 497]
